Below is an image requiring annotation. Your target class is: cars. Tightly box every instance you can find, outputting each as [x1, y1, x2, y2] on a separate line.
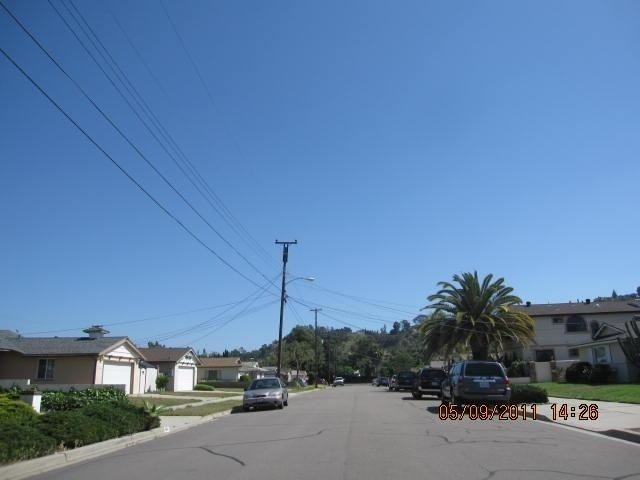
[440, 360, 512, 410]
[388, 370, 415, 392]
[243, 377, 289, 411]
[333, 377, 344, 386]
[412, 368, 445, 399]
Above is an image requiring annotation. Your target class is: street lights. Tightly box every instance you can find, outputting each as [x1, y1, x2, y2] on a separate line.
[276, 278, 314, 377]
